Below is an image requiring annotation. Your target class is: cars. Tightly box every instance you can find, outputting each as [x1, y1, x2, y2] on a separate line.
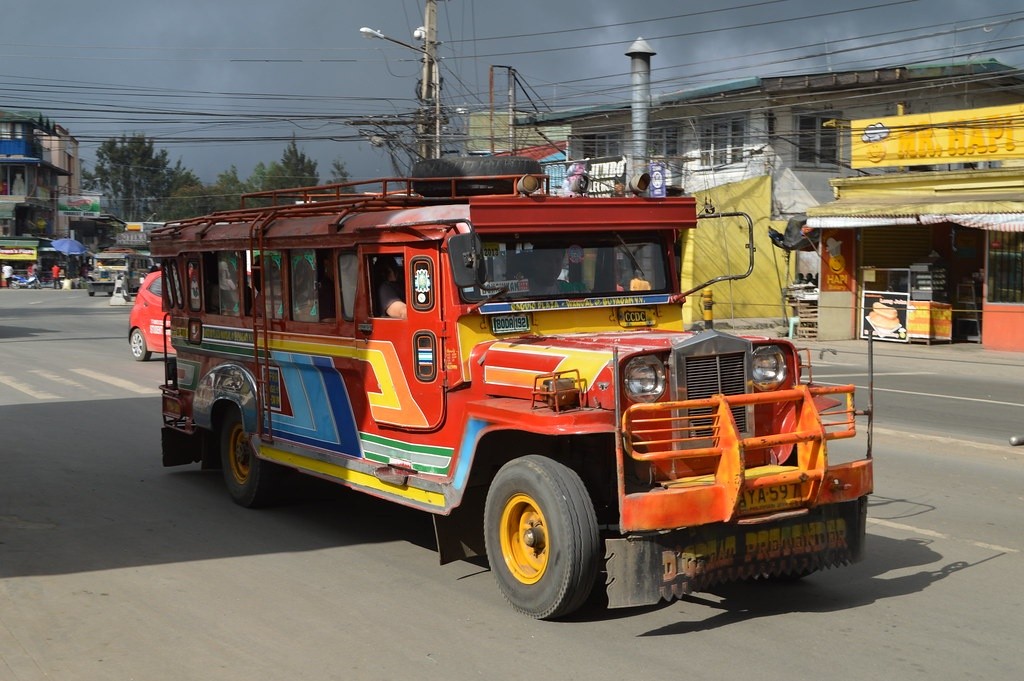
[128, 270, 179, 361]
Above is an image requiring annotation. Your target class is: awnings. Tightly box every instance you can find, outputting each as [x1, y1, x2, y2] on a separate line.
[782, 214, 820, 252]
[805, 192, 1024, 232]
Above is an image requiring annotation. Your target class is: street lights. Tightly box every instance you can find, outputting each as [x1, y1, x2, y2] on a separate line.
[360, 27, 440, 159]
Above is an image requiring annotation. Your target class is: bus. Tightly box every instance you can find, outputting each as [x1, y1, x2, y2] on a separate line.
[146, 156, 874, 621]
[87, 249, 154, 296]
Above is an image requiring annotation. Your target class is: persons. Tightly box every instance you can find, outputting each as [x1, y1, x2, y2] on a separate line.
[506, 238, 580, 298]
[218, 254, 237, 315]
[2, 263, 62, 289]
[79, 259, 108, 280]
[617, 269, 651, 292]
[210, 273, 219, 314]
[243, 254, 407, 322]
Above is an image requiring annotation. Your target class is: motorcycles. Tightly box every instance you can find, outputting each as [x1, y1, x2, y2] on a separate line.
[11, 272, 42, 289]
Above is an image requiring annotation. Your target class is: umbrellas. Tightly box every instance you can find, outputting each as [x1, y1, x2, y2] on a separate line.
[50, 238, 88, 256]
